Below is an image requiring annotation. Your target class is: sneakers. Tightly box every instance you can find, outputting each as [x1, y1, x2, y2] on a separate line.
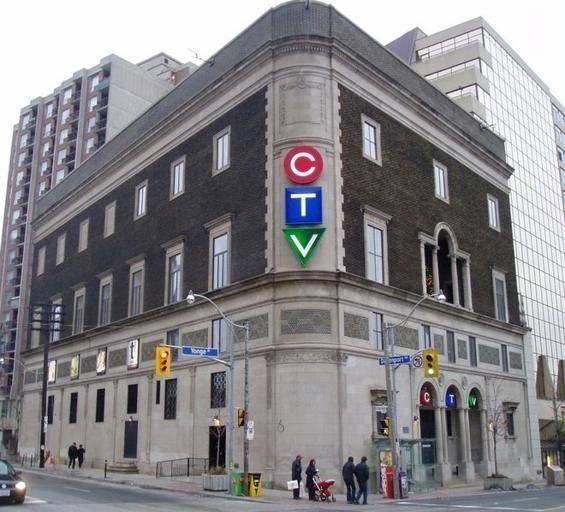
[347, 501, 368, 504]
[293, 497, 317, 500]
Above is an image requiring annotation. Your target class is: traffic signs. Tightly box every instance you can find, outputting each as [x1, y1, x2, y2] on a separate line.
[380, 355, 410, 364]
[184, 347, 218, 358]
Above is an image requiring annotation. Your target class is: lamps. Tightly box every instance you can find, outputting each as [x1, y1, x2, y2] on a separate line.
[212, 415, 219, 421]
[128, 415, 133, 423]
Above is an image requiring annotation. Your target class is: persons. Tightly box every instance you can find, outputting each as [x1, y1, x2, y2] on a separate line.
[355, 456, 371, 505]
[342, 457, 357, 503]
[68, 443, 77, 469]
[306, 459, 317, 500]
[77, 445, 85, 468]
[291, 456, 302, 499]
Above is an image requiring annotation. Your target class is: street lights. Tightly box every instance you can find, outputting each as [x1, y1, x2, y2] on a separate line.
[0, 356, 46, 466]
[379, 287, 448, 500]
[188, 290, 250, 492]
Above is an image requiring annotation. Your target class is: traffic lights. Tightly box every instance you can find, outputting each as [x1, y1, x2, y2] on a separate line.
[239, 409, 246, 425]
[159, 350, 169, 374]
[427, 354, 434, 376]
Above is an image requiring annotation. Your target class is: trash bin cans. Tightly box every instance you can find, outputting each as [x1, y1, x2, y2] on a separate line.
[231, 472, 263, 497]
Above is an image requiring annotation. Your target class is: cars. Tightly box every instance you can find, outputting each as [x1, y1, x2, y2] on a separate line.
[0, 458, 27, 505]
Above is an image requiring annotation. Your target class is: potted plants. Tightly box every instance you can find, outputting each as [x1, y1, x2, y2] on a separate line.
[201, 464, 230, 492]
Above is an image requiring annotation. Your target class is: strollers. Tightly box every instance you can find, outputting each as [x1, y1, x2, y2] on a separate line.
[312, 474, 336, 502]
